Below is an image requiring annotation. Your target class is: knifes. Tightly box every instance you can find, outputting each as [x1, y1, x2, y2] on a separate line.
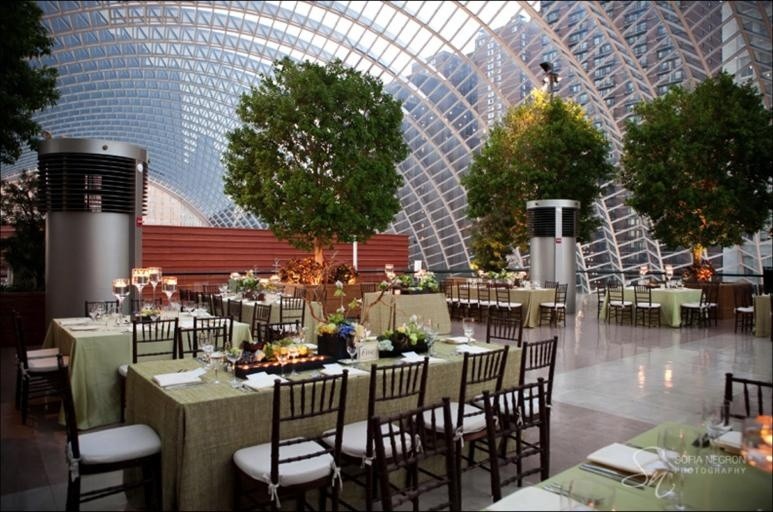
[579, 460, 656, 490]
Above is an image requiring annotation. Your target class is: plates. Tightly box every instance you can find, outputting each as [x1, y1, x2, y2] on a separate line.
[128, 323, 154, 332]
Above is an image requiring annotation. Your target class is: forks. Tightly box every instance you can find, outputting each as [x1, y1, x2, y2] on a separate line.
[233, 383, 253, 393]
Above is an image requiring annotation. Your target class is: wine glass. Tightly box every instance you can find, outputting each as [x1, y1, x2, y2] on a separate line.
[95, 300, 107, 320]
[655, 425, 702, 511]
[276, 349, 291, 380]
[701, 395, 735, 468]
[198, 302, 209, 317]
[198, 332, 216, 369]
[217, 282, 228, 293]
[346, 336, 360, 370]
[462, 317, 475, 346]
[209, 351, 224, 384]
[182, 299, 195, 317]
[225, 342, 242, 383]
[88, 305, 98, 323]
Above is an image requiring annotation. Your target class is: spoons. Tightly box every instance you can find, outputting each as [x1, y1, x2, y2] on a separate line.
[542, 482, 605, 507]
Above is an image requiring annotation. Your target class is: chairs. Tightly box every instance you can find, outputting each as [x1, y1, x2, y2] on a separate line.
[17, 276, 773, 511]
